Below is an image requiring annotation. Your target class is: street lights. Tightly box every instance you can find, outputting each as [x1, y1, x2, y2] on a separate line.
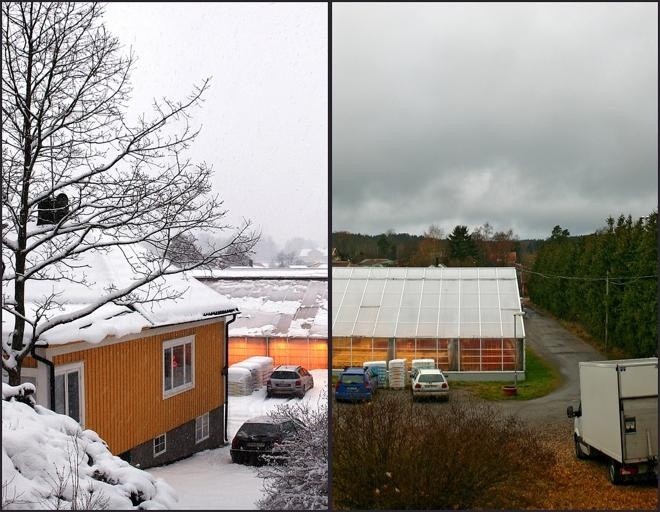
[513, 311, 526, 386]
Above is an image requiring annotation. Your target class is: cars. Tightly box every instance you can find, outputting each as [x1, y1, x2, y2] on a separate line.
[230, 416, 305, 467]
[410, 366, 449, 401]
[268, 364, 313, 399]
[335, 365, 377, 401]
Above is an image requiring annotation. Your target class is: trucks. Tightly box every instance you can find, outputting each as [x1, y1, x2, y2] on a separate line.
[567, 357, 659, 485]
